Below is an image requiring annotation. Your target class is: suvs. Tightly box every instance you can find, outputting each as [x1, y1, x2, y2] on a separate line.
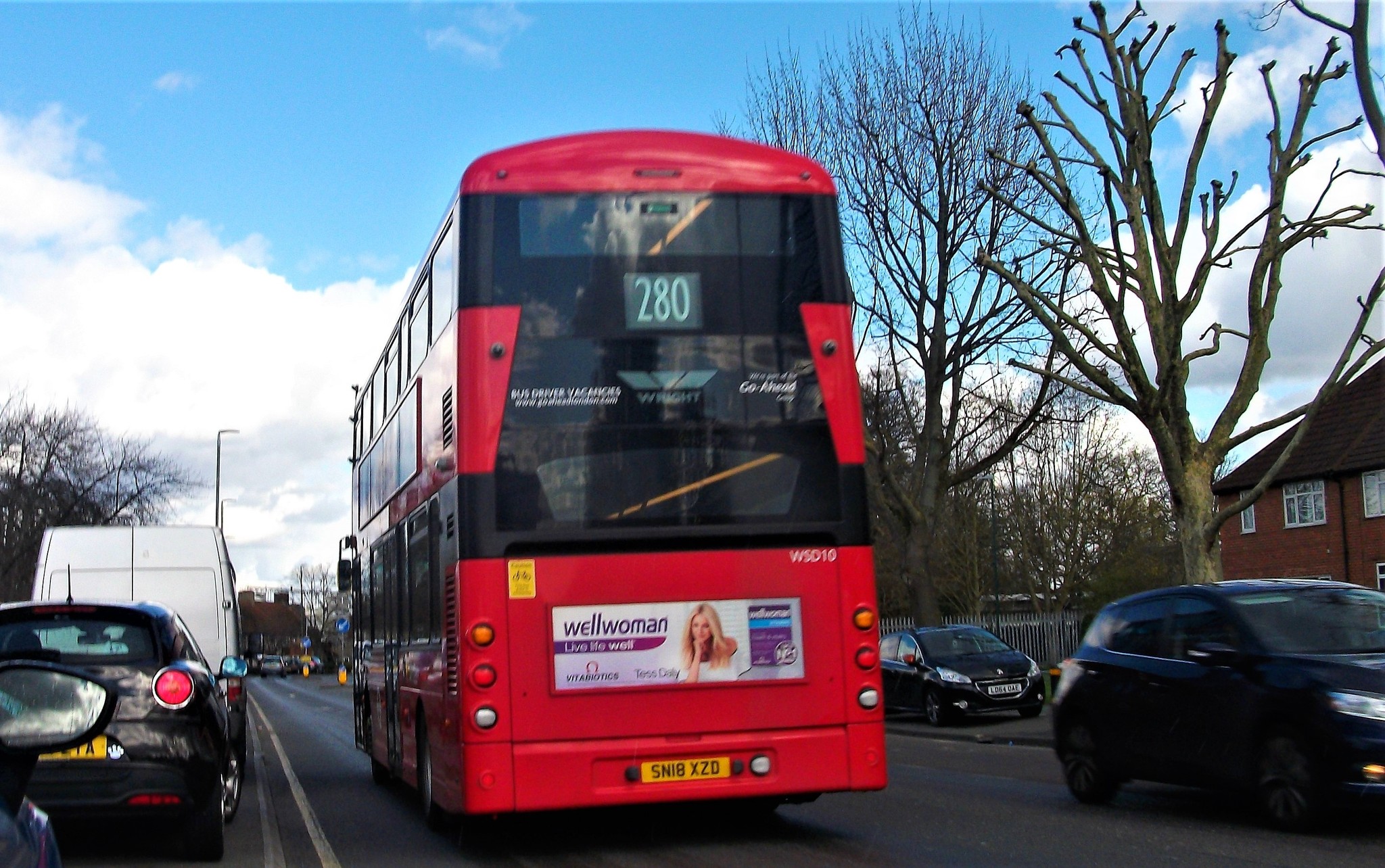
[0, 599, 249, 862]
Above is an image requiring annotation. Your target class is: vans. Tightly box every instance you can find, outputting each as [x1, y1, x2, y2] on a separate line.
[30, 524, 247, 765]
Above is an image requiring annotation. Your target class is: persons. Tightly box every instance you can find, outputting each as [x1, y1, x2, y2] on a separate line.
[678, 603, 752, 683]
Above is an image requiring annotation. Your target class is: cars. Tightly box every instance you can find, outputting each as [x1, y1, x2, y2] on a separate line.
[245, 653, 325, 678]
[1050, 578, 1385, 833]
[878, 623, 1046, 727]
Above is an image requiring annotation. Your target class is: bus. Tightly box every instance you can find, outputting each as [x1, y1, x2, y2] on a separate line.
[336, 127, 889, 831]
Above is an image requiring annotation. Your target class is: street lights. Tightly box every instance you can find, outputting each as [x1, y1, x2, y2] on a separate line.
[979, 474, 1000, 633]
[221, 498, 237, 533]
[215, 428, 240, 527]
